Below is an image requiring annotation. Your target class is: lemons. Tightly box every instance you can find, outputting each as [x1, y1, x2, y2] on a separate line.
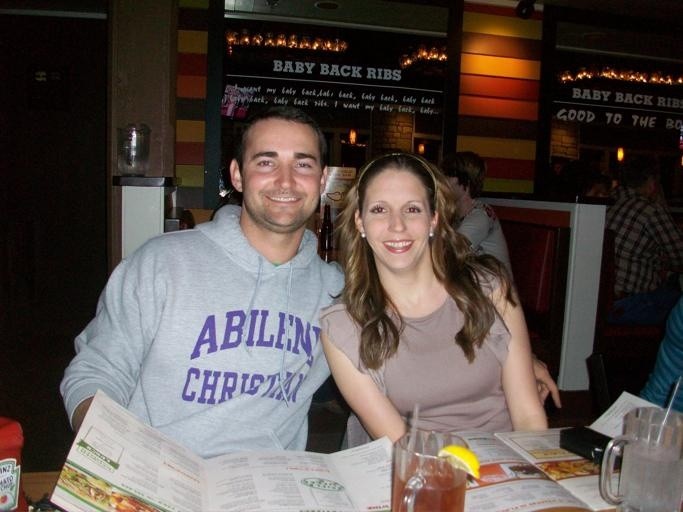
[437, 444, 481, 480]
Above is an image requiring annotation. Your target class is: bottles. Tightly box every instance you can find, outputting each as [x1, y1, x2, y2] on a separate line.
[321, 205, 333, 251]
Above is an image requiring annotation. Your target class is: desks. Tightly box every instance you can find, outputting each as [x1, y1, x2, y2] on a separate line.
[2, 458, 683, 512]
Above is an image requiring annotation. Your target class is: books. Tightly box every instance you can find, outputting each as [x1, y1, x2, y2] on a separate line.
[446, 392, 664, 511]
[50, 386, 396, 512]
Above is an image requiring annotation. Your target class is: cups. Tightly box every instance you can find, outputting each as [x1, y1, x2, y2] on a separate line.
[393, 432, 470, 507]
[600, 407, 683, 507]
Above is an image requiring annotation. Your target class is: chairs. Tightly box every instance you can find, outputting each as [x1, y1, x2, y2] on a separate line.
[591, 303, 668, 386]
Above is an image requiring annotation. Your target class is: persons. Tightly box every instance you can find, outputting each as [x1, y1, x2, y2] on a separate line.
[605, 151, 683, 326]
[319, 153, 548, 444]
[59, 107, 562, 454]
[638, 293, 683, 415]
[439, 149, 514, 278]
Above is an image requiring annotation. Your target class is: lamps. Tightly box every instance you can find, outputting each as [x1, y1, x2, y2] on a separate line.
[228, 30, 348, 57]
[559, 66, 683, 87]
[397, 44, 451, 68]
